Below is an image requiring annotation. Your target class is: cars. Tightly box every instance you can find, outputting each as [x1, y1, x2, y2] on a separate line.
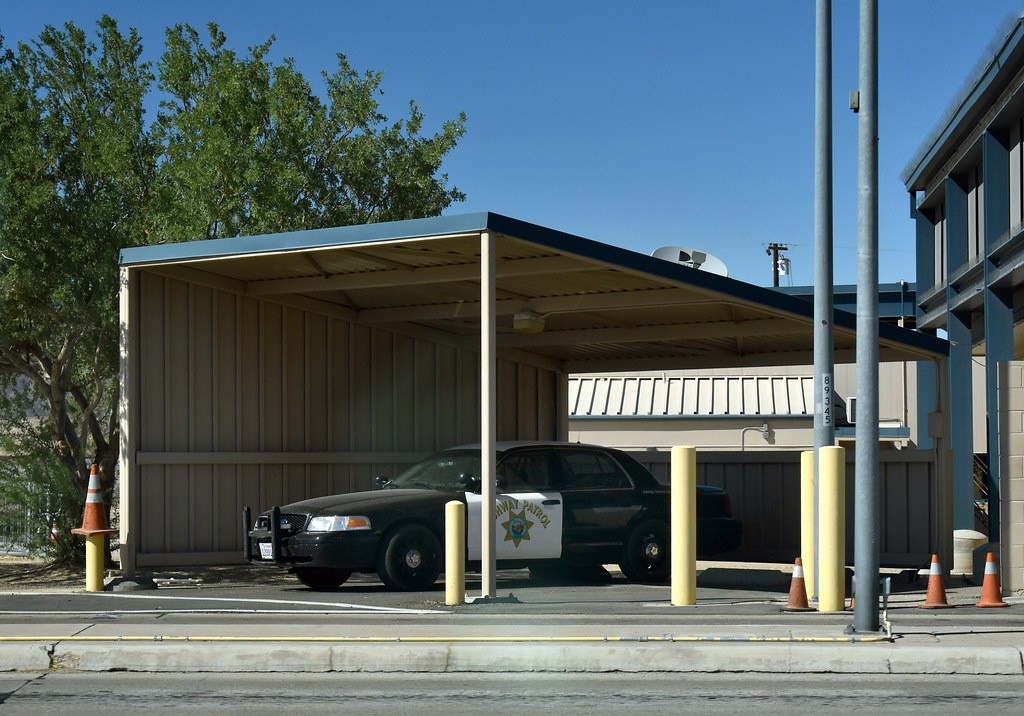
[244, 440, 741, 591]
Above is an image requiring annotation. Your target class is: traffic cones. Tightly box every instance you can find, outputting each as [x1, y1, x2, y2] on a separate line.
[975, 552, 1008, 607]
[918, 553, 955, 609]
[781, 557, 817, 612]
[71, 463, 120, 536]
